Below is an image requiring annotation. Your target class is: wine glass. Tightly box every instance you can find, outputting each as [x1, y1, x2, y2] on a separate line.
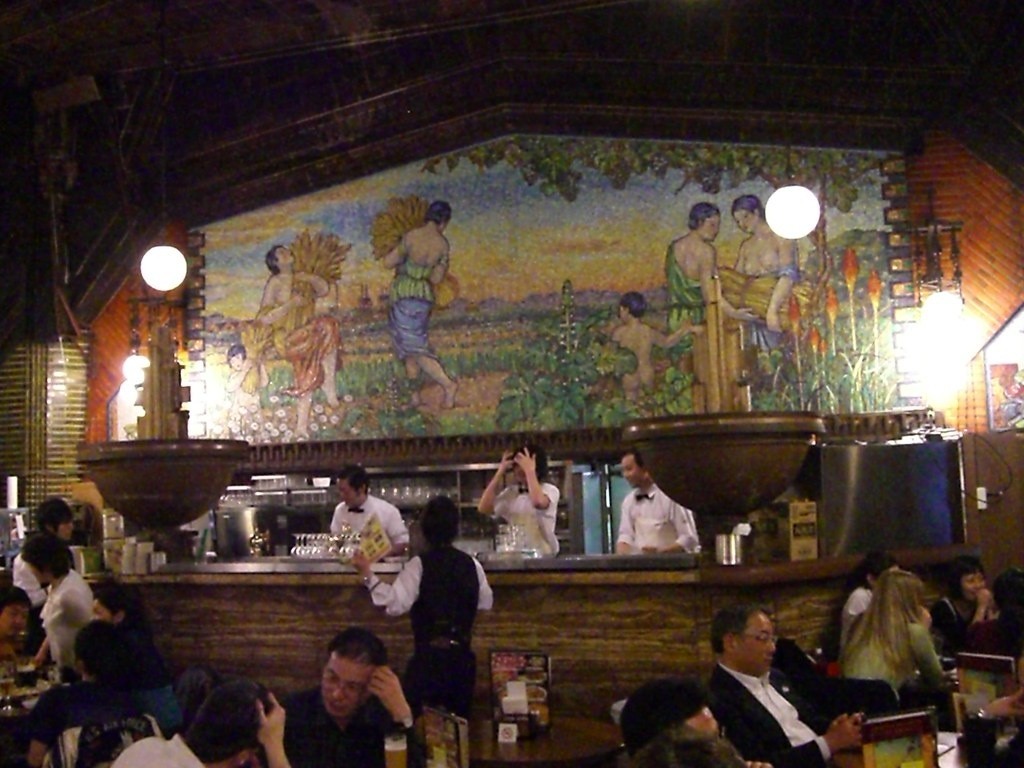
[495, 523, 531, 551]
[290, 532, 361, 556]
[0, 661, 16, 711]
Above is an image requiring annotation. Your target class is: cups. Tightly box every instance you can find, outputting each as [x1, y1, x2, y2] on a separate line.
[81, 546, 100, 574]
[383, 734, 407, 768]
[367, 478, 456, 502]
[101, 509, 168, 575]
[714, 533, 744, 565]
[220, 475, 334, 506]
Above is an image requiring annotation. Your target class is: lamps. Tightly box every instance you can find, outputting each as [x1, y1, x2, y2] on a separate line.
[764, 80, 821, 240]
[141, 118, 187, 291]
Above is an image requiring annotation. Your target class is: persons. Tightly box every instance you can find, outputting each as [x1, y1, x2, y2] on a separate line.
[621, 547, 1024, 768]
[616, 449, 700, 555]
[328, 465, 408, 560]
[354, 495, 496, 725]
[0, 499, 425, 768]
[477, 445, 561, 559]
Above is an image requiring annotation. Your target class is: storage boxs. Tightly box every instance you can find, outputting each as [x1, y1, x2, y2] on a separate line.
[757, 501, 818, 563]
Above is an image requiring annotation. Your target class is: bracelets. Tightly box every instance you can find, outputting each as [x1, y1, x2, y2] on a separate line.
[976, 707, 996, 720]
[976, 607, 986, 614]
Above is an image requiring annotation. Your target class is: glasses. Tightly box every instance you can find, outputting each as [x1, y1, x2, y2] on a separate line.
[747, 632, 778, 644]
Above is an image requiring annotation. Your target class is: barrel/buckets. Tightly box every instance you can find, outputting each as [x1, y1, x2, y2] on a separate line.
[214, 507, 272, 558]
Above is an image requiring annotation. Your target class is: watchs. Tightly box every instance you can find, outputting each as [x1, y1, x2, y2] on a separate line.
[396, 712, 416, 731]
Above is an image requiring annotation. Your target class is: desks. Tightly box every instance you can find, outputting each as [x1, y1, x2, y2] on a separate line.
[468, 717, 626, 768]
[833, 732, 1014, 768]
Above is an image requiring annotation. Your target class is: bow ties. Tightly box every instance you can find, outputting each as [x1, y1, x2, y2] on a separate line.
[348, 507, 364, 513]
[635, 494, 654, 501]
[518, 488, 529, 494]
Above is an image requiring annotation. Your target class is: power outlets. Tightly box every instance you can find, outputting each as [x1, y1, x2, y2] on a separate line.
[977, 487, 990, 510]
